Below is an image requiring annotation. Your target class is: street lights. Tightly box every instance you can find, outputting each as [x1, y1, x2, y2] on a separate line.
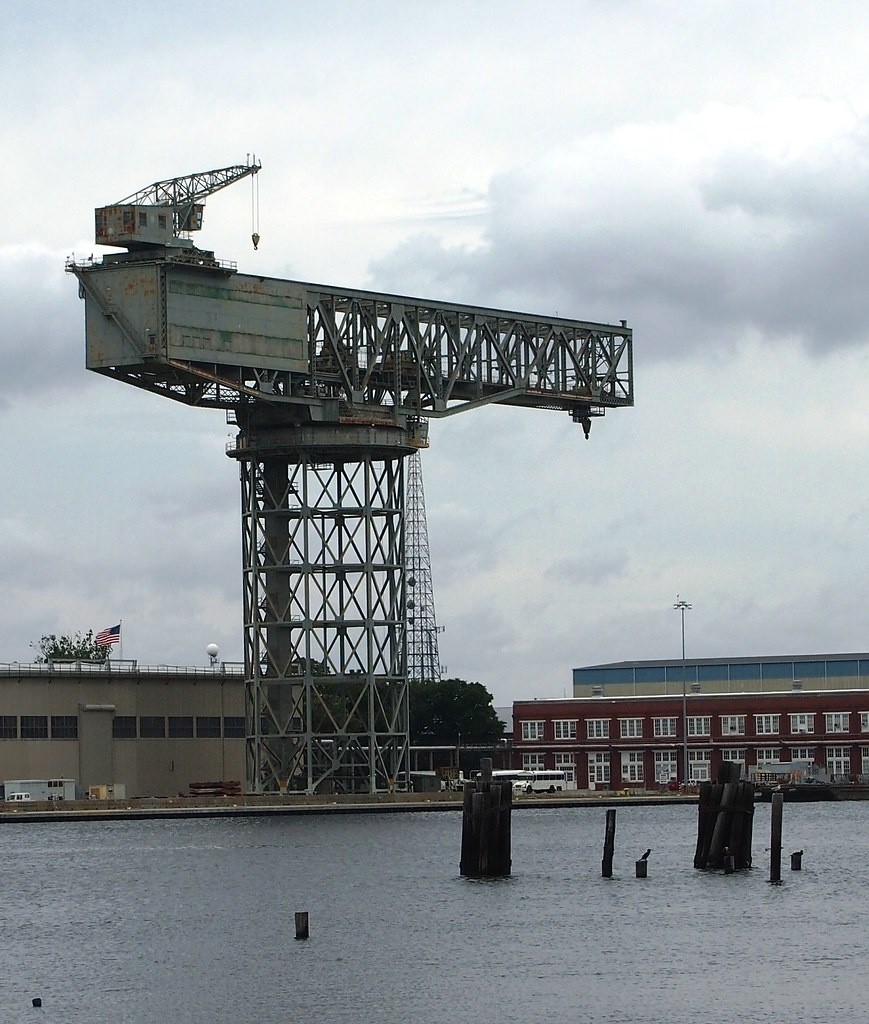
[673, 594, 692, 797]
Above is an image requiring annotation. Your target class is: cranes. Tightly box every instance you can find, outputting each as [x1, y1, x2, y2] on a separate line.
[93, 151, 263, 273]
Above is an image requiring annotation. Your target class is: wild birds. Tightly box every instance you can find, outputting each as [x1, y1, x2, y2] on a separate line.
[721, 846, 732, 857]
[639, 848, 652, 861]
[789, 850, 803, 856]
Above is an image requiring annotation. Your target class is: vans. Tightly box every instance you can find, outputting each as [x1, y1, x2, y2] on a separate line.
[6, 793, 31, 801]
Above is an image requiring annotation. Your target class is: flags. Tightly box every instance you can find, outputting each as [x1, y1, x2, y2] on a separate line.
[97, 624, 120, 647]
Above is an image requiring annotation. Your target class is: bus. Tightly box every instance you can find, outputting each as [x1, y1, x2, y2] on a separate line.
[678, 779, 710, 791]
[476, 770, 567, 794]
